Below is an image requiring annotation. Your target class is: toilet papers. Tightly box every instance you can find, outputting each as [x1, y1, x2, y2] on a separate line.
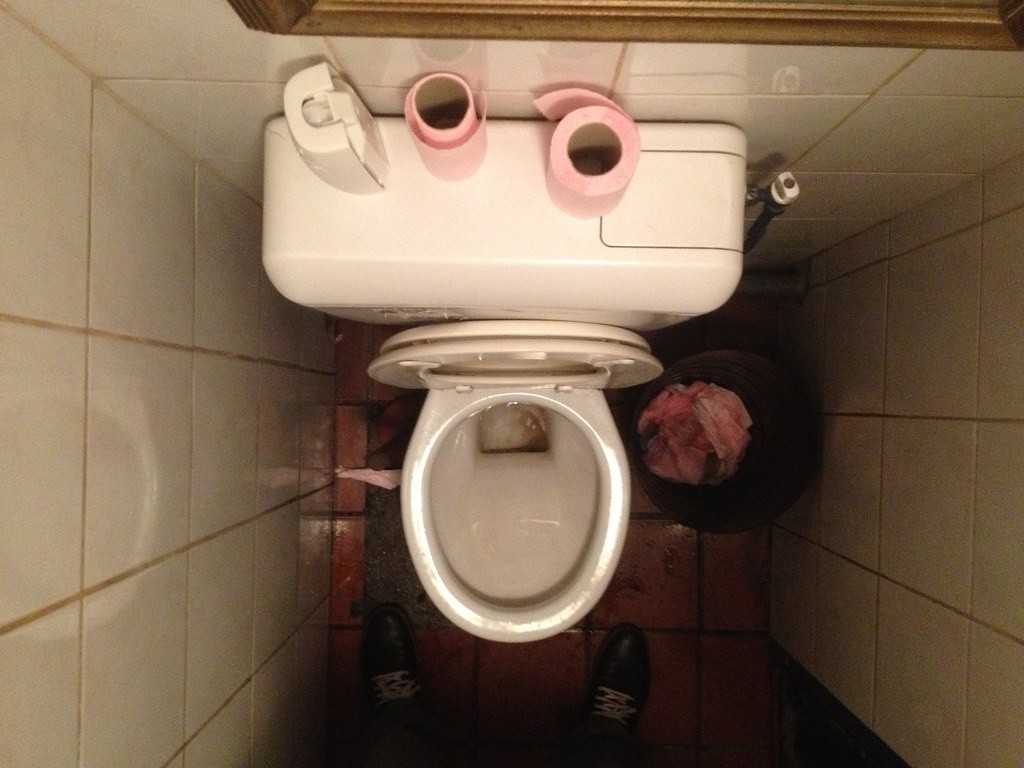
[531, 87, 642, 221]
[403, 72, 489, 183]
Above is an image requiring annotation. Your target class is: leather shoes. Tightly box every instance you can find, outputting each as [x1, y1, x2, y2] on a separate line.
[586, 623, 651, 733]
[362, 603, 421, 713]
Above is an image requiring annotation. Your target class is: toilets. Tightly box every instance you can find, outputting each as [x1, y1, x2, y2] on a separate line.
[256, 114, 750, 645]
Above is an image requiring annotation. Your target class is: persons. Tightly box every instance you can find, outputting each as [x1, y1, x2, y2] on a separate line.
[359, 604, 651, 768]
